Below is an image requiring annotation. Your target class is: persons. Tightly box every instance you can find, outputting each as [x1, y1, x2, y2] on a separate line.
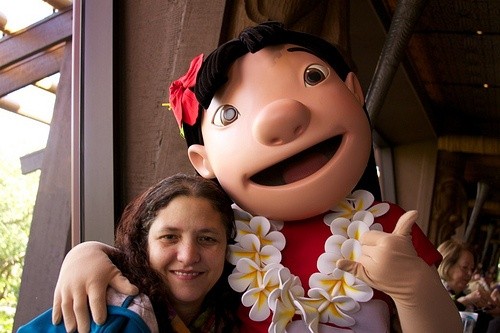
[17, 173, 239, 333]
[52, 20, 463, 333]
[427, 238, 500, 333]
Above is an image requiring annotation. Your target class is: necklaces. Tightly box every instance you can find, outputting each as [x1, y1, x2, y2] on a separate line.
[228, 189, 390, 333]
